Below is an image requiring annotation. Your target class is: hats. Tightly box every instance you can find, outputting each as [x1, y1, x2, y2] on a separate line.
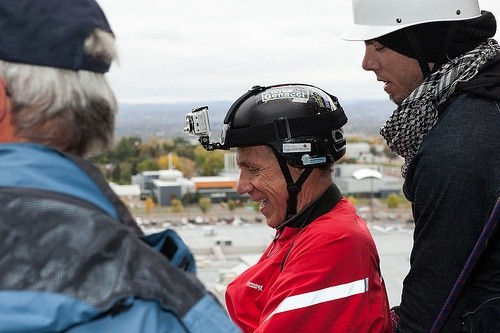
[375, 10, 497, 64]
[0, 0, 113, 73]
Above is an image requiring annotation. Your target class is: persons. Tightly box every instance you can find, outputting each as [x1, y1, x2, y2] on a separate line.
[223, 82, 393, 333]
[1, 0, 244, 333]
[341, 0, 500, 333]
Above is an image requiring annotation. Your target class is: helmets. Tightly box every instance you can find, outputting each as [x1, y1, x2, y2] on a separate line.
[342, 0, 482, 41]
[221, 84, 348, 167]
